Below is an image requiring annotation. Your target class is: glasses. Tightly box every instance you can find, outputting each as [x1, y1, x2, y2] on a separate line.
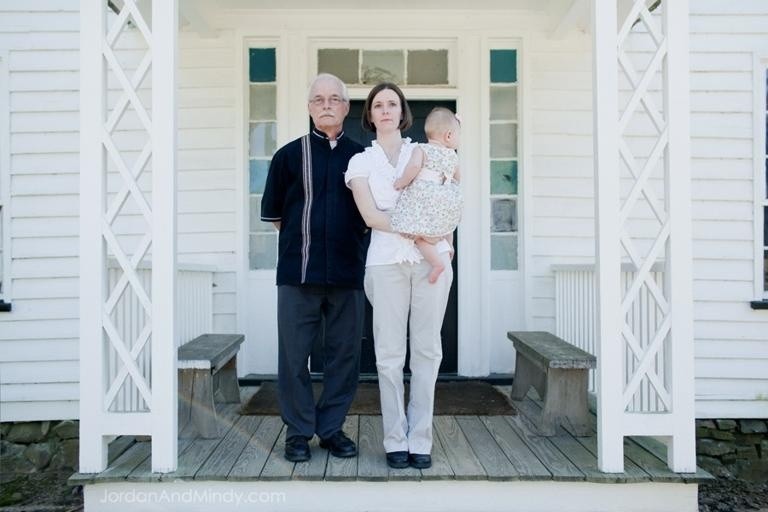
[309, 94, 347, 105]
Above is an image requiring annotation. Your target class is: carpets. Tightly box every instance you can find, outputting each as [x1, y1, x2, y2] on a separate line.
[235, 378, 519, 417]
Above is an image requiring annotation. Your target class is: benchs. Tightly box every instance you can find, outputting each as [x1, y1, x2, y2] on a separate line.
[507, 330, 597, 437]
[178, 333, 245, 439]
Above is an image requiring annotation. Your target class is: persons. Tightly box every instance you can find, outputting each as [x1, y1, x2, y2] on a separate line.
[342, 81, 454, 471]
[256, 70, 375, 465]
[388, 106, 467, 284]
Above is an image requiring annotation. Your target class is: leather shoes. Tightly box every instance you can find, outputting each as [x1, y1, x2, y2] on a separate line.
[410, 451, 435, 470]
[284, 435, 314, 464]
[385, 449, 410, 469]
[320, 428, 362, 461]
